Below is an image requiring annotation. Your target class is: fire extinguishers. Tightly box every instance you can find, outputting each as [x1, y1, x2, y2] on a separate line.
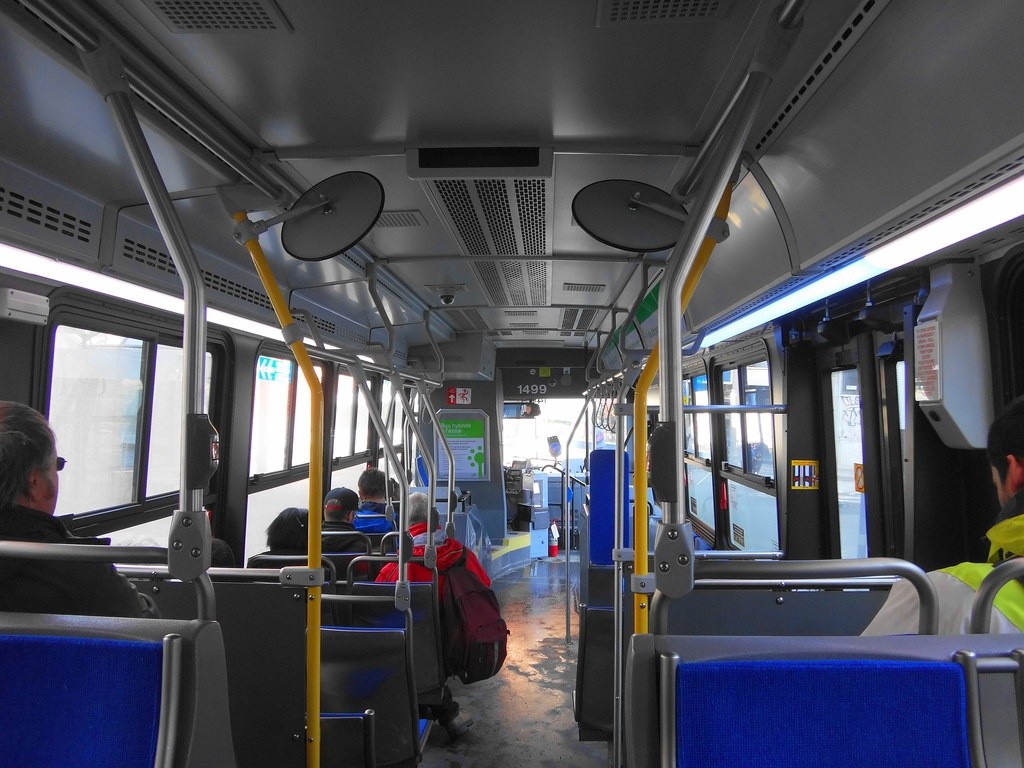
[548, 518, 559, 557]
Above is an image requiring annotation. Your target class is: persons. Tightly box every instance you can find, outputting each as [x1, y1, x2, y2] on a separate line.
[247, 507, 325, 568]
[521, 405, 532, 417]
[1, 397, 160, 620]
[375, 492, 492, 724]
[854, 401, 1023, 639]
[354, 467, 401, 537]
[321, 488, 372, 581]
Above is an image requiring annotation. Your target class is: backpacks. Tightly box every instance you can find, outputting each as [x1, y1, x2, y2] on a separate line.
[413, 546, 510, 684]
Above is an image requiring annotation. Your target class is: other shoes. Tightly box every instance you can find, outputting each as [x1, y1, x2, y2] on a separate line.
[445, 710, 473, 740]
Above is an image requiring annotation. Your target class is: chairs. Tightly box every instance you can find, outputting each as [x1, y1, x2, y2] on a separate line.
[0, 456, 1024, 768]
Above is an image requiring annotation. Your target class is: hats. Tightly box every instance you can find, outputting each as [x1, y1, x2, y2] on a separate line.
[324, 487, 363, 513]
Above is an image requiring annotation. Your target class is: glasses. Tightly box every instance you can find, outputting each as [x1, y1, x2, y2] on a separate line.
[48, 457, 67, 471]
